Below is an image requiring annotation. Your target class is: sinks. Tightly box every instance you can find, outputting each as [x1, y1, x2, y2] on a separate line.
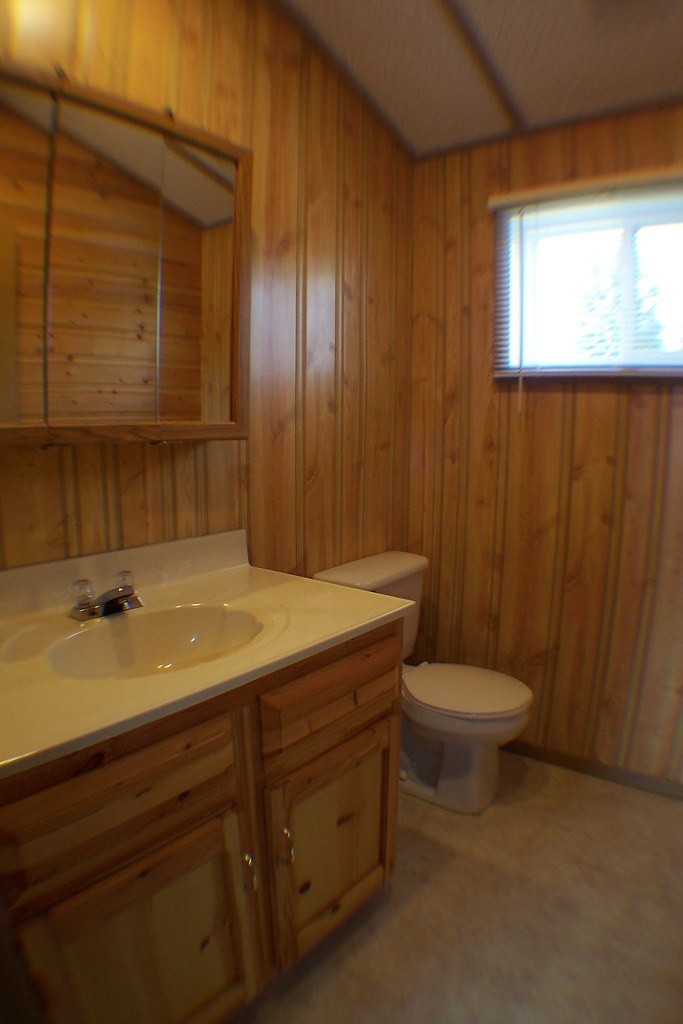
[46, 603, 265, 681]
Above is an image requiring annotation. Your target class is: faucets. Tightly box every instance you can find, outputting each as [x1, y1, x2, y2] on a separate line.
[93, 584, 135, 617]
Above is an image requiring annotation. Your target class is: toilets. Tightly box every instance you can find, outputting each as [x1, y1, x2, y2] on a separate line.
[312, 549, 535, 816]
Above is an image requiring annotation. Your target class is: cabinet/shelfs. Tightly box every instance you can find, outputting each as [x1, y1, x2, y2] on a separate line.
[0, 616, 408, 1024]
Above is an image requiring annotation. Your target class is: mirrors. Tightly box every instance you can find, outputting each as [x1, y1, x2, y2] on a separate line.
[0, 58, 254, 446]
